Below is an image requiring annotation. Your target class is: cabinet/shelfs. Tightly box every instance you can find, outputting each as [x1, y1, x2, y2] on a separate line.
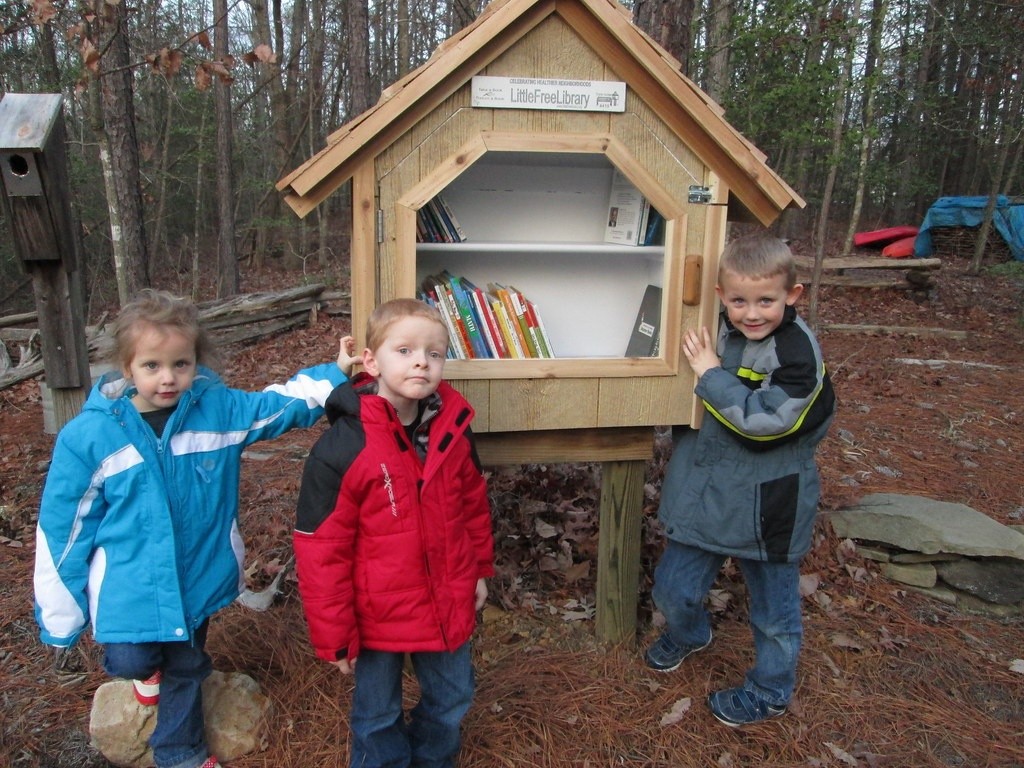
[275, 1, 807, 644]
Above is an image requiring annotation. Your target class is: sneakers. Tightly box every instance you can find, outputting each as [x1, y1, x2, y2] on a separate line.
[132, 668, 161, 705]
[198, 753, 221, 768]
[708, 687, 787, 727]
[644, 624, 712, 672]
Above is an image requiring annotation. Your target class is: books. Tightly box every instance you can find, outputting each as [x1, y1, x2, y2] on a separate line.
[416, 190, 467, 243]
[416, 269, 557, 360]
[623, 284, 662, 357]
[603, 165, 662, 247]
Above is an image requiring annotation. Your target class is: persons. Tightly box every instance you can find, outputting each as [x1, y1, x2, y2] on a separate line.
[643, 234, 836, 728]
[34, 289, 365, 768]
[293, 298, 495, 768]
